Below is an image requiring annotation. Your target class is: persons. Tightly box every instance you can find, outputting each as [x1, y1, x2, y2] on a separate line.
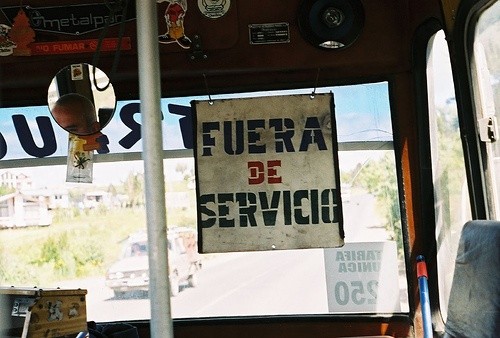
[130, 243, 145, 255]
[51, 92, 102, 134]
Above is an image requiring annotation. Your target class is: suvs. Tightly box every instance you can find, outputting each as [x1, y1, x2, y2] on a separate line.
[106, 230, 197, 296]
